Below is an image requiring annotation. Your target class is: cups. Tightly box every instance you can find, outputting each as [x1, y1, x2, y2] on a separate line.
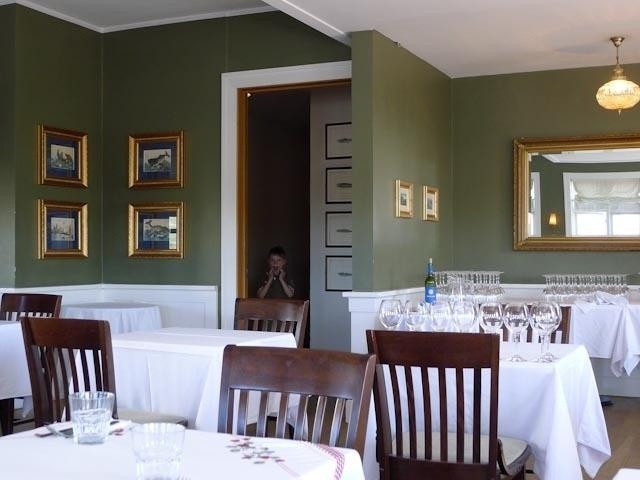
[69, 391, 113, 444]
[132, 422, 184, 480]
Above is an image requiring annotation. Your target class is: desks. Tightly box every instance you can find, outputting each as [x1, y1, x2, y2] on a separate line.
[362, 343, 612, 480]
[411, 289, 640, 405]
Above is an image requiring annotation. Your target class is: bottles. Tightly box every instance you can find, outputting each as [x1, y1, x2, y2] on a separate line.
[425, 258, 438, 303]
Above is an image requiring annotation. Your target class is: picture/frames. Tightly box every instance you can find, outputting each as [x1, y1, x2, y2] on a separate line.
[395, 178, 414, 219]
[126, 198, 184, 259]
[127, 128, 186, 191]
[38, 122, 91, 190]
[324, 120, 354, 291]
[421, 184, 440, 222]
[37, 199, 89, 260]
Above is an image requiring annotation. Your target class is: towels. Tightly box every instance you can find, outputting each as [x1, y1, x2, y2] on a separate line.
[582, 291, 630, 306]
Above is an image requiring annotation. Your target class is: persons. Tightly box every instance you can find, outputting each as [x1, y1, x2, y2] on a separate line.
[250, 244, 299, 298]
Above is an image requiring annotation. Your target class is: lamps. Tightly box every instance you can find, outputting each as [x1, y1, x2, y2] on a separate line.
[596, 34, 640, 116]
[548, 212, 557, 233]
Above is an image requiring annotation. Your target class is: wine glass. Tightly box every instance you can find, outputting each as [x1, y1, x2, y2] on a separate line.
[538, 275, 628, 301]
[433, 270, 506, 298]
[379, 298, 563, 363]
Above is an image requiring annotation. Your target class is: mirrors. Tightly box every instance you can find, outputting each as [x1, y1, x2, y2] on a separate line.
[512, 130, 640, 251]
